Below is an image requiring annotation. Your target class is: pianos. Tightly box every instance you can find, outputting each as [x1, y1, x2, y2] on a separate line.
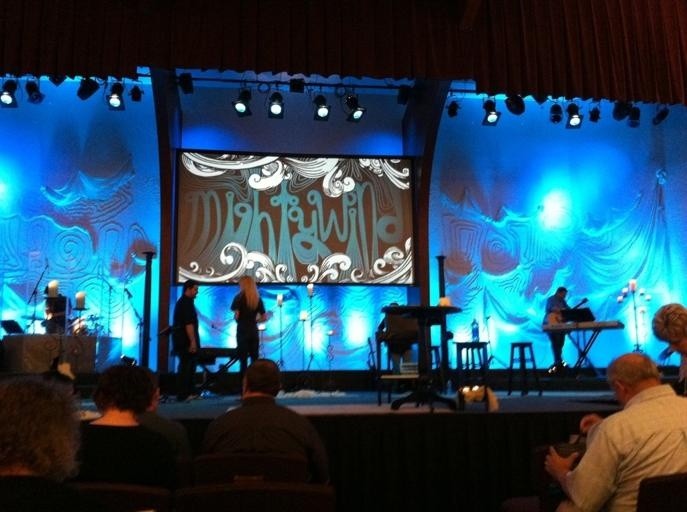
[543, 321, 624, 331]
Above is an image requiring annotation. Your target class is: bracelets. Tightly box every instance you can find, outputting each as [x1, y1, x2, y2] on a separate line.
[258, 318, 261, 321]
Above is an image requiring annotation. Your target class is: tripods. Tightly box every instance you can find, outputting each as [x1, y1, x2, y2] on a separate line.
[278, 306, 287, 368]
[485, 316, 507, 369]
[307, 296, 321, 371]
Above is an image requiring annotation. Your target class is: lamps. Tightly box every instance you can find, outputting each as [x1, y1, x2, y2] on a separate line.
[0, 77, 669, 130]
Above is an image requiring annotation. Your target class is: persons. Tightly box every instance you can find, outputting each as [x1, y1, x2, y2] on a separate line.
[229, 276, 268, 393]
[497, 351, 686, 512]
[650, 302, 687, 387]
[37, 358, 331, 488]
[1, 377, 80, 494]
[540, 287, 574, 365]
[170, 280, 205, 402]
[377, 303, 412, 368]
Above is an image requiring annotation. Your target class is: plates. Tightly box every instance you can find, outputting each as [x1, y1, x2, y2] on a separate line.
[75, 410, 101, 422]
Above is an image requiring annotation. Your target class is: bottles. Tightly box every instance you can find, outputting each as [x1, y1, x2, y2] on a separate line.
[471, 317, 479, 342]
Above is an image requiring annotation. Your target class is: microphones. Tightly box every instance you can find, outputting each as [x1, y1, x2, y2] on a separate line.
[124, 288, 132, 297]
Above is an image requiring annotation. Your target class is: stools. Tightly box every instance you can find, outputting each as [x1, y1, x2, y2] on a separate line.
[507, 342, 544, 396]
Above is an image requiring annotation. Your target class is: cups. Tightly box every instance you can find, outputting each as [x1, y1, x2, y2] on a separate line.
[556, 442, 577, 458]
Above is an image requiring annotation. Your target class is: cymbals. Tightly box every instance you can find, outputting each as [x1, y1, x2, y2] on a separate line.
[22, 316, 43, 321]
